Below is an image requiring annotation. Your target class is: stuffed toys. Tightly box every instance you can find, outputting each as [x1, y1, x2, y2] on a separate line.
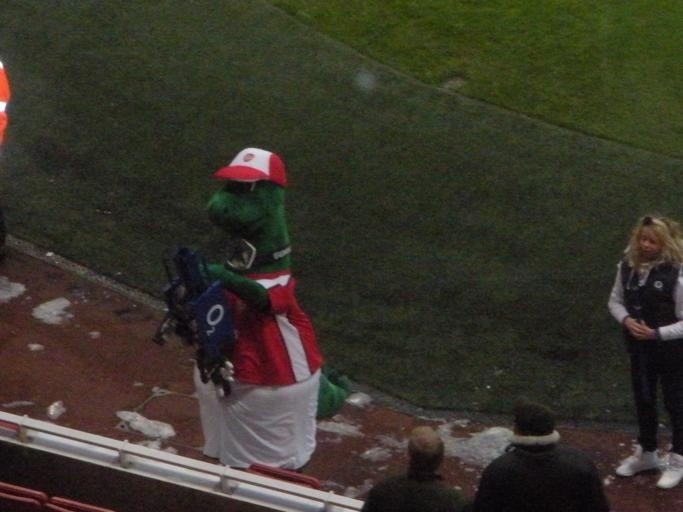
[194, 148, 352, 474]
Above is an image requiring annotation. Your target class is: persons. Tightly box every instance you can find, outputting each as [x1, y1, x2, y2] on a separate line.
[1, 57, 12, 265]
[360, 425, 472, 510]
[608, 212, 683, 489]
[472, 402, 611, 510]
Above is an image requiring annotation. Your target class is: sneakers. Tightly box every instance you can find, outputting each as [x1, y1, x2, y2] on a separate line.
[656, 454, 683, 490]
[615, 446, 660, 478]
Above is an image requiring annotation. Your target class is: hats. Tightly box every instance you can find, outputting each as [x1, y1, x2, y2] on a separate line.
[211, 146, 287, 189]
